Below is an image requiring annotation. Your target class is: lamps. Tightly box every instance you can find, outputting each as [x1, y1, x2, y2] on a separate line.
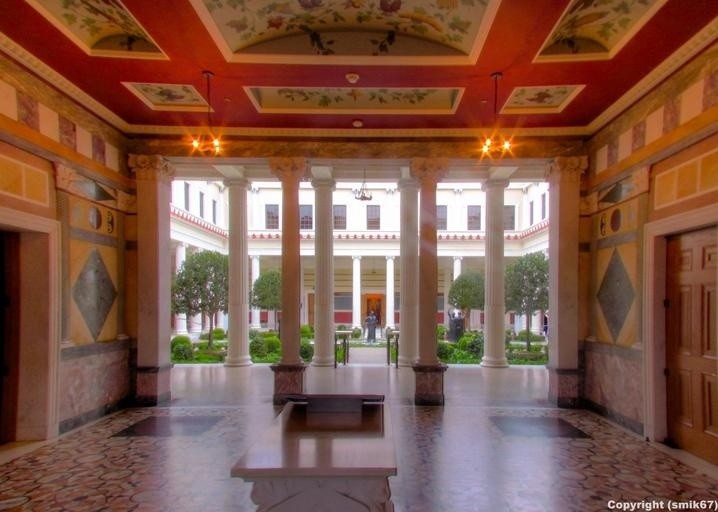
[353, 166, 373, 201]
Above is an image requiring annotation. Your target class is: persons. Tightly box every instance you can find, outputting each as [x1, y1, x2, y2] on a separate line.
[365, 310, 378, 343]
[452, 311, 463, 339]
[447, 305, 455, 341]
[542, 309, 548, 342]
